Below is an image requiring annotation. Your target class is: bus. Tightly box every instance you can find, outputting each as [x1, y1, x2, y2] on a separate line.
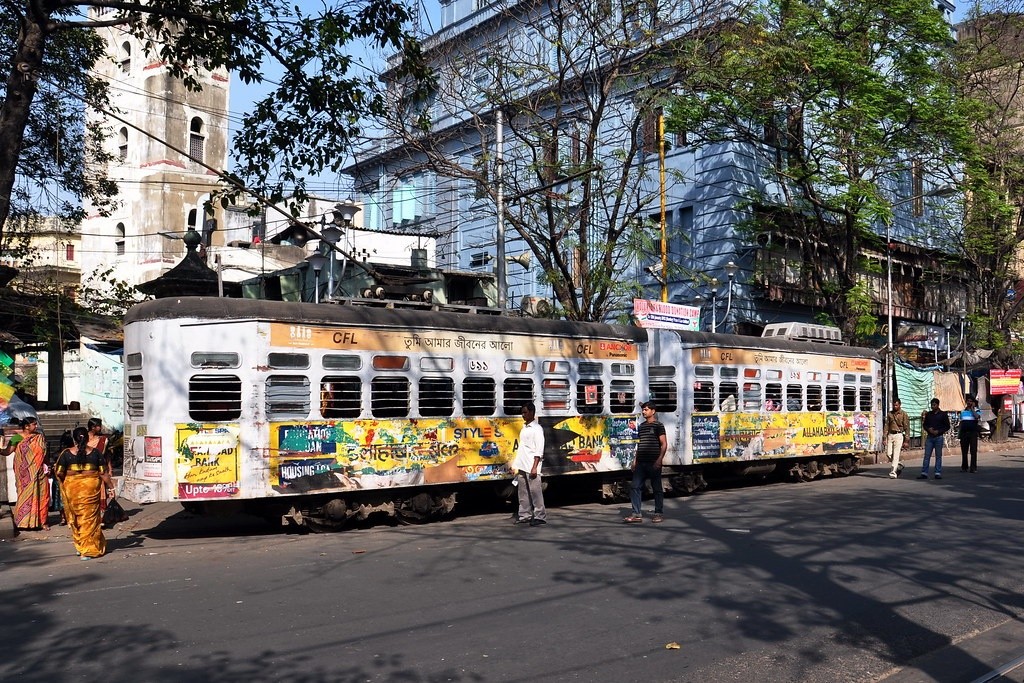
[116, 296, 884, 534]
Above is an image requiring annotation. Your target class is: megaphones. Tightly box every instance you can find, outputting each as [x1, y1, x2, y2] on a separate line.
[505, 249, 532, 271]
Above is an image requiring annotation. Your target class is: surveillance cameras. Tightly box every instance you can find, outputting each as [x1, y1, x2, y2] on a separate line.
[643, 262, 664, 274]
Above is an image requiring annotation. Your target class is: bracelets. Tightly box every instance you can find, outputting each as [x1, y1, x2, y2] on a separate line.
[107, 488, 115, 492]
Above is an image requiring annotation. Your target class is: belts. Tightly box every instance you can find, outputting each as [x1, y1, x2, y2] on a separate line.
[890, 431, 901, 434]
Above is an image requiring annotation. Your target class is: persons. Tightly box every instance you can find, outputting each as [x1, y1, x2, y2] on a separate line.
[917, 398, 951, 480]
[978, 420, 991, 435]
[53, 427, 116, 560]
[52, 418, 114, 527]
[883, 398, 910, 479]
[623, 400, 668, 524]
[959, 395, 981, 473]
[510, 401, 547, 526]
[0, 417, 51, 532]
[720, 392, 801, 413]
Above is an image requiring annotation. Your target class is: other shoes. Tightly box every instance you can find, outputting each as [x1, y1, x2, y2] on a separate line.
[515, 519, 528, 524]
[960, 469, 967, 472]
[890, 475, 895, 479]
[651, 516, 664, 523]
[970, 469, 977, 473]
[76, 552, 92, 561]
[59, 517, 67, 526]
[935, 475, 942, 479]
[916, 474, 927, 479]
[529, 520, 546, 527]
[897, 465, 905, 476]
[622, 514, 643, 522]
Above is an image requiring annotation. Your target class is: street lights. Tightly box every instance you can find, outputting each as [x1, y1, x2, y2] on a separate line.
[885, 187, 958, 412]
